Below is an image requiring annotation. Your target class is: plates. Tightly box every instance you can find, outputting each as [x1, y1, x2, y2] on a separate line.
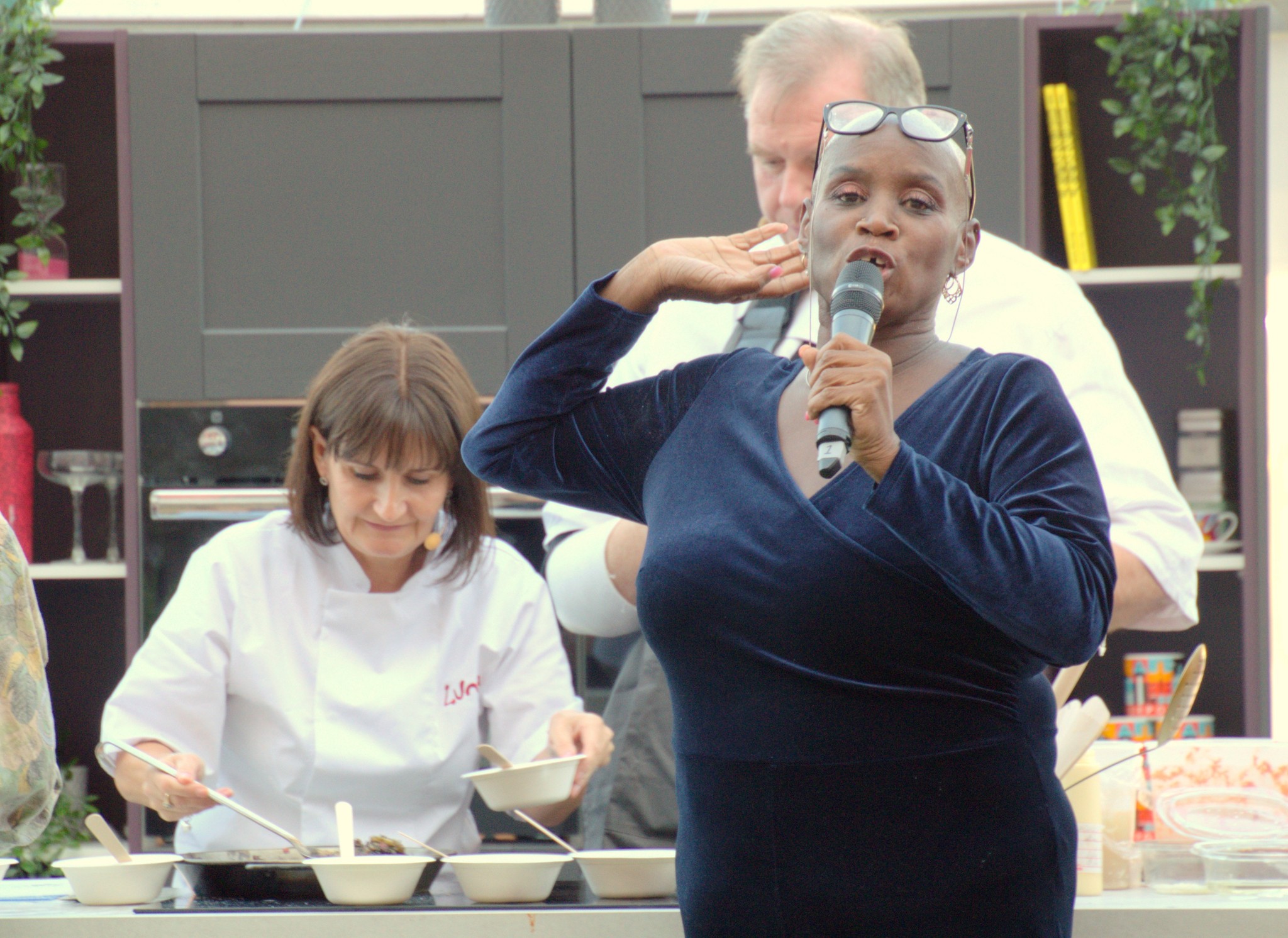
[1201, 539, 1244, 554]
[1, 859, 20, 891]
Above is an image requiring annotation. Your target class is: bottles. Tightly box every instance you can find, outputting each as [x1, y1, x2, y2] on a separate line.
[0, 381, 36, 565]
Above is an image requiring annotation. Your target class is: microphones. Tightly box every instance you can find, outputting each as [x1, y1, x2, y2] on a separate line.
[815, 261, 886, 479]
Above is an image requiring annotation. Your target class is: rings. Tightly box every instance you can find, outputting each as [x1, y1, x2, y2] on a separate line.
[162, 793, 173, 809]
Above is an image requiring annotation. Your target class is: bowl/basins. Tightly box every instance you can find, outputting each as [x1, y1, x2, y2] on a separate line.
[461, 755, 590, 810]
[301, 855, 436, 906]
[1128, 841, 1288, 900]
[438, 853, 574, 903]
[51, 853, 184, 905]
[567, 848, 676, 899]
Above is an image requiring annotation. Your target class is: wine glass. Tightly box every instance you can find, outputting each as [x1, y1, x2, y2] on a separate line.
[36, 450, 123, 565]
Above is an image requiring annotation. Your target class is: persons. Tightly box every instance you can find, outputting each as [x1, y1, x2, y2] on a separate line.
[461, 96, 1117, 938]
[539, 7, 1206, 846]
[96, 323, 616, 896]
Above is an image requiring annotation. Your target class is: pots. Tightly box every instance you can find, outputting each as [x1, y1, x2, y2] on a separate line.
[174, 845, 457, 898]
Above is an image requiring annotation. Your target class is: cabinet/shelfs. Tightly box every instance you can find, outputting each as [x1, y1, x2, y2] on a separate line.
[1026, 7, 1274, 741]
[128, 15, 1024, 405]
[0, 22, 144, 858]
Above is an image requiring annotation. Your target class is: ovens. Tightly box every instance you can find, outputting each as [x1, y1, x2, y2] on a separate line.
[137, 399, 551, 649]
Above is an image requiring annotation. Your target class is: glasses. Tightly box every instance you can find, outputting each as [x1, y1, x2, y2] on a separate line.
[805, 100, 976, 242]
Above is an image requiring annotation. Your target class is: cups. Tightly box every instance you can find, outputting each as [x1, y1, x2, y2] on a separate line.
[1193, 511, 1238, 542]
[1097, 653, 1214, 739]
